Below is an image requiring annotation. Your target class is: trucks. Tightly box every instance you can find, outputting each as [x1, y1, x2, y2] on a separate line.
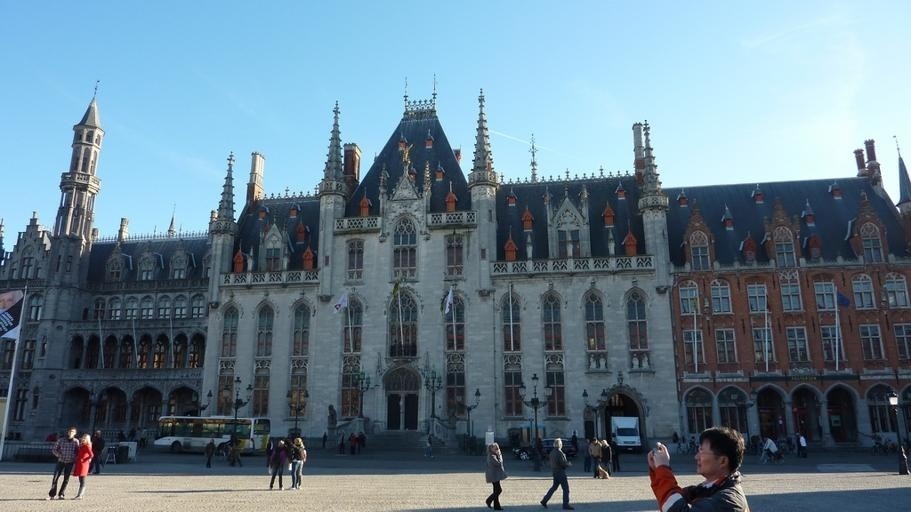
[606, 415, 642, 454]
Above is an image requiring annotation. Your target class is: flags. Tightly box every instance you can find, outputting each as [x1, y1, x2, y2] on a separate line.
[445, 288, 452, 313]
[837, 291, 850, 306]
[767, 295, 771, 312]
[332, 293, 347, 314]
[0, 290, 25, 339]
[694, 298, 698, 313]
[393, 283, 398, 298]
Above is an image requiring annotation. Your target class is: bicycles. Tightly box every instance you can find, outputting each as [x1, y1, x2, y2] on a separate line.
[760, 449, 787, 466]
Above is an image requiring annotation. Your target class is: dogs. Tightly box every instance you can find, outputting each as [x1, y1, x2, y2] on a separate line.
[598, 464, 609, 479]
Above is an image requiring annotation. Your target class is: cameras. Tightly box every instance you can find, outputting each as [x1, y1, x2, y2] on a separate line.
[653, 447, 663, 453]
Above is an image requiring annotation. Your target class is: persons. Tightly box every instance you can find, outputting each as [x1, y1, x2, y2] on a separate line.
[583, 436, 620, 479]
[540, 438, 575, 509]
[795, 432, 809, 458]
[205, 439, 215, 468]
[485, 443, 507, 510]
[647, 427, 751, 512]
[764, 435, 778, 461]
[47, 426, 147, 500]
[424, 431, 434, 458]
[267, 438, 307, 491]
[323, 432, 366, 454]
[231, 440, 245, 467]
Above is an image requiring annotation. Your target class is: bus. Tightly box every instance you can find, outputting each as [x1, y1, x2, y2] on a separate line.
[154, 414, 271, 456]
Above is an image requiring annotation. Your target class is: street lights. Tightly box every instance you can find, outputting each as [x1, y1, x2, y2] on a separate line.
[223, 376, 254, 442]
[424, 368, 445, 420]
[352, 370, 372, 417]
[285, 377, 312, 439]
[730, 389, 759, 449]
[518, 373, 554, 448]
[191, 389, 212, 416]
[582, 386, 609, 442]
[886, 389, 911, 474]
[456, 388, 482, 451]
[88, 388, 108, 441]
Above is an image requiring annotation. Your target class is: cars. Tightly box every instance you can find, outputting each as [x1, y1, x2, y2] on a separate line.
[513, 437, 577, 460]
